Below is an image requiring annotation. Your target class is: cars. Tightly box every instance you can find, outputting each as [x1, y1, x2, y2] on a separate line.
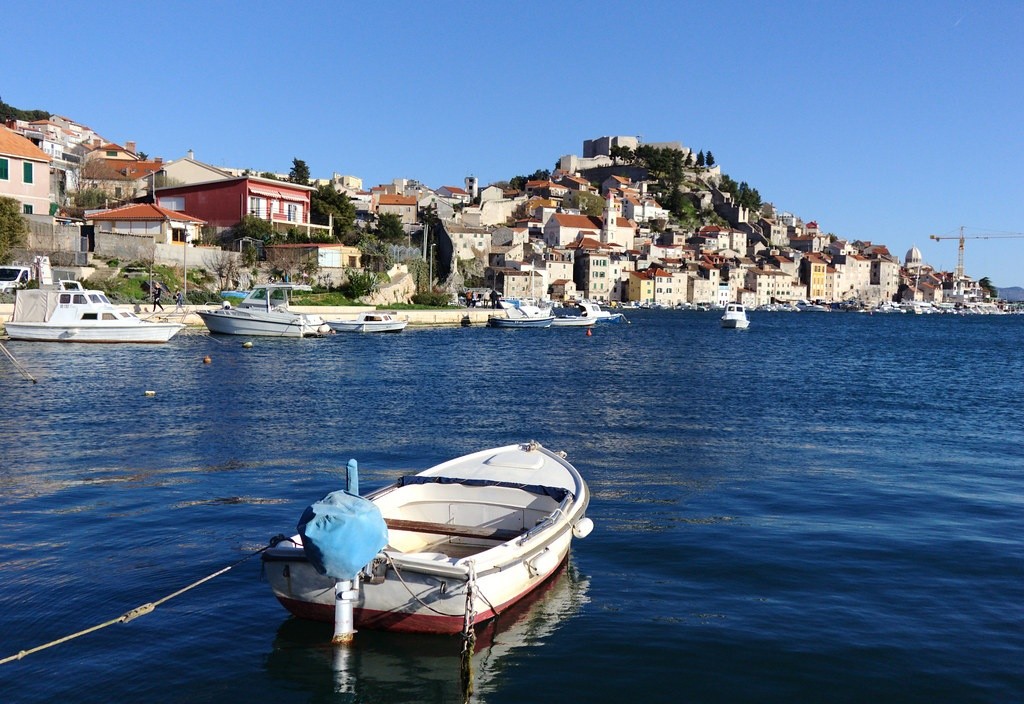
[624, 301, 715, 311]
[0, 266, 32, 292]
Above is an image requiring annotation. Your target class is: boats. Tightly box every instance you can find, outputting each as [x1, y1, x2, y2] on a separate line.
[261, 439, 593, 641]
[319, 314, 409, 333]
[756, 302, 868, 313]
[196, 284, 333, 337]
[488, 316, 555, 328]
[3, 280, 187, 342]
[552, 316, 597, 326]
[593, 304, 621, 320]
[878, 305, 1022, 316]
[719, 302, 750, 328]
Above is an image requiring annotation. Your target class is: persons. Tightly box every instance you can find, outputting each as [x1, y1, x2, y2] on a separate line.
[483, 290, 490, 308]
[490, 290, 497, 309]
[152, 282, 163, 312]
[175, 289, 184, 313]
[465, 289, 478, 308]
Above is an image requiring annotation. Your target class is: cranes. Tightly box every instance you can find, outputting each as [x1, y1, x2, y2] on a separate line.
[929, 224, 1024, 278]
